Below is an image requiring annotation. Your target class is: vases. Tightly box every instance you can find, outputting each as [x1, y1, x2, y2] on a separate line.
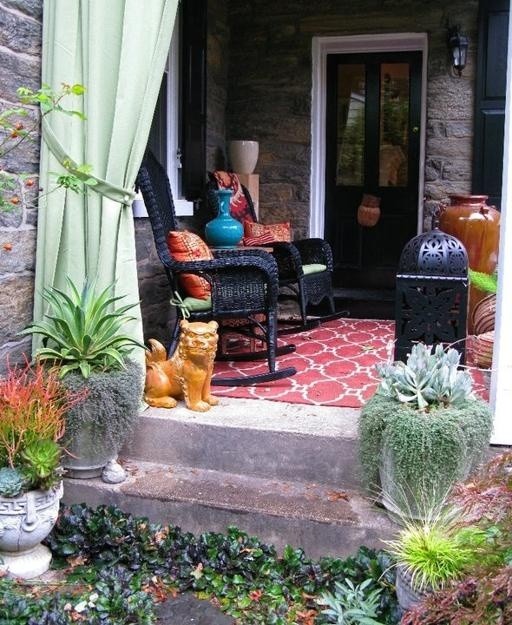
[432, 194, 500, 330]
[206, 189, 243, 249]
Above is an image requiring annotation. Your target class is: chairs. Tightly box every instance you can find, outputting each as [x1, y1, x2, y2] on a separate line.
[136, 147, 297, 386]
[205, 179, 351, 334]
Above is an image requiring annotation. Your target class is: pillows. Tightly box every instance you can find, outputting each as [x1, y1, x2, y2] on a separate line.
[171, 228, 217, 299]
[245, 220, 291, 241]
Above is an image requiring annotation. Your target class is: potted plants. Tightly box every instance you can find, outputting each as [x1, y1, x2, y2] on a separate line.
[369, 472, 482, 612]
[357, 344, 492, 525]
[1, 354, 91, 577]
[15, 278, 141, 479]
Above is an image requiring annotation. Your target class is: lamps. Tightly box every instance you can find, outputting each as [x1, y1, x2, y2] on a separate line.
[445, 19, 469, 77]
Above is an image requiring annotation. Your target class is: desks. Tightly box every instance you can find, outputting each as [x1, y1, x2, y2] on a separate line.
[203, 245, 274, 352]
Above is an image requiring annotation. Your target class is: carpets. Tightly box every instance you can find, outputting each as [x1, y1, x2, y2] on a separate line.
[207, 317, 396, 408]
